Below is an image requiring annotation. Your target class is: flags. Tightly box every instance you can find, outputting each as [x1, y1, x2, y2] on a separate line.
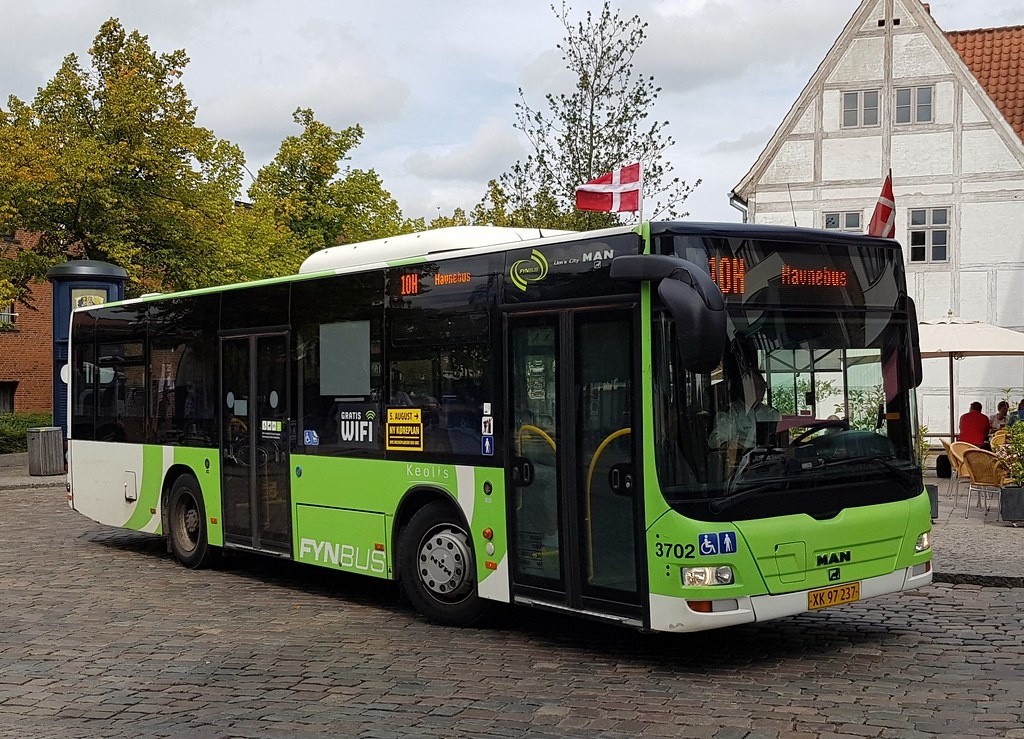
[869, 176, 896, 240]
[575, 162, 643, 212]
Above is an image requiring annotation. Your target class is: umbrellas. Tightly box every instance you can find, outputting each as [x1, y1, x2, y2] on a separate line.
[838, 309, 1023, 441]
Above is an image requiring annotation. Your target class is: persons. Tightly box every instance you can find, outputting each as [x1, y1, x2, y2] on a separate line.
[823, 415, 843, 435]
[989, 401, 1017, 442]
[958, 402, 991, 451]
[707, 370, 794, 467]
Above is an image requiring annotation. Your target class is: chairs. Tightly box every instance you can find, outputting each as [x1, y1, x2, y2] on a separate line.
[436, 397, 481, 432]
[939, 426, 1017, 522]
[702, 380, 739, 469]
[78, 387, 109, 417]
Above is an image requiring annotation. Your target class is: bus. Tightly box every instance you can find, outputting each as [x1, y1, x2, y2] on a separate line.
[64, 224, 934, 635]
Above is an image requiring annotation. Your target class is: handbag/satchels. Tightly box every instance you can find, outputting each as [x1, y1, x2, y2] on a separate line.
[936, 454, 951, 478]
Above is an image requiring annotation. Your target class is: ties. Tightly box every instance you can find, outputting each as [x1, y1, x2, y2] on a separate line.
[754, 412, 757, 423]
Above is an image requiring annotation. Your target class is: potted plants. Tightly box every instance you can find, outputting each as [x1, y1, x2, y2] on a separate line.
[995, 386, 1024, 522]
[916, 426, 938, 518]
